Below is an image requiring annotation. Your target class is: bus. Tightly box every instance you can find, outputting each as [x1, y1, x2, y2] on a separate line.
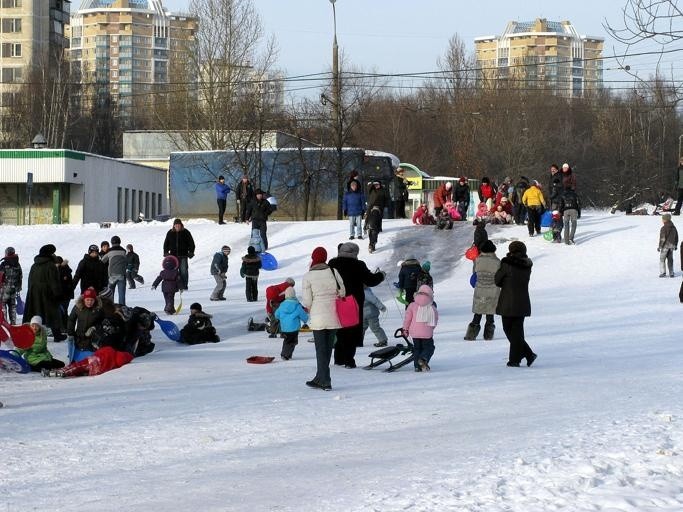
[166, 144, 409, 220]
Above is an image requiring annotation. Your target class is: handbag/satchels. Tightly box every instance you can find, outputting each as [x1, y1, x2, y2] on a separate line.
[336, 296, 359, 328]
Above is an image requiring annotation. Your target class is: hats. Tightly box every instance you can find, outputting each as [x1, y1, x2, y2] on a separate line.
[40, 244, 56, 256]
[221, 246, 231, 251]
[662, 214, 671, 221]
[5, 247, 15, 256]
[89, 245, 99, 255]
[30, 316, 42, 324]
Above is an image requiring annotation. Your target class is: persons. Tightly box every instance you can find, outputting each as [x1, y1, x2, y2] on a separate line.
[672, 156, 682, 215]
[657, 214, 677, 278]
[163, 218, 194, 290]
[248, 229, 264, 253]
[240, 246, 262, 302]
[300, 247, 346, 392]
[340, 163, 584, 245]
[209, 245, 230, 301]
[235, 176, 252, 224]
[247, 277, 308, 361]
[215, 176, 229, 225]
[493, 241, 537, 367]
[679, 241, 682, 307]
[328, 242, 387, 368]
[0, 235, 156, 377]
[151, 255, 220, 344]
[463, 240, 501, 341]
[397, 252, 439, 371]
[244, 188, 271, 249]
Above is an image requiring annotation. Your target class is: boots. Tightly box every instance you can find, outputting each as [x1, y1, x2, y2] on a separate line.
[464, 322, 480, 340]
[483, 323, 494, 340]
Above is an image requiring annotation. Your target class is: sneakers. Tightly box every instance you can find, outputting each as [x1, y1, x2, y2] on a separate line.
[670, 275, 674, 277]
[374, 340, 388, 347]
[219, 297, 226, 300]
[529, 234, 534, 237]
[527, 353, 537, 366]
[368, 245, 373, 254]
[358, 236, 362, 239]
[507, 360, 520, 366]
[373, 245, 375, 251]
[318, 385, 332, 391]
[659, 273, 666, 277]
[346, 363, 356, 368]
[349, 236, 354, 239]
[569, 239, 575, 244]
[210, 298, 218, 301]
[415, 367, 422, 372]
[418, 358, 430, 372]
[306, 380, 320, 387]
[53, 334, 67, 342]
[40, 368, 50, 377]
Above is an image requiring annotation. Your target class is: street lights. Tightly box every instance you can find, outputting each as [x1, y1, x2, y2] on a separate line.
[329, 0, 339, 147]
